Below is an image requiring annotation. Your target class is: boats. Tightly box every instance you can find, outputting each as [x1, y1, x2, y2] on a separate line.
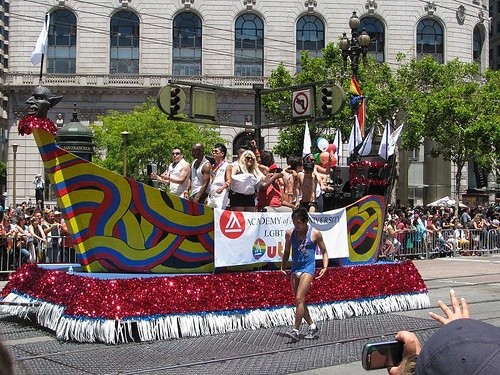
[17, 81, 398, 272]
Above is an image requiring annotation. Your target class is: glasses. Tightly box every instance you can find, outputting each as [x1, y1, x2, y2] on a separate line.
[172, 153, 180, 155]
[6, 220, 12, 222]
[310, 159, 316, 162]
[245, 157, 253, 161]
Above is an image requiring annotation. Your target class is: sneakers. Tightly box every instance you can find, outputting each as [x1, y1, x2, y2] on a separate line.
[304, 326, 319, 338]
[286, 331, 299, 340]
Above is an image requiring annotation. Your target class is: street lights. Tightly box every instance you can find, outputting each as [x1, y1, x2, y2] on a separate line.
[12, 142, 18, 208]
[340, 10, 370, 117]
[121, 130, 130, 178]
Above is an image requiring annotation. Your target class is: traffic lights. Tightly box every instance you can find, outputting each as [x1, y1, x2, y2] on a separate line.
[157, 85, 186, 114]
[317, 84, 346, 116]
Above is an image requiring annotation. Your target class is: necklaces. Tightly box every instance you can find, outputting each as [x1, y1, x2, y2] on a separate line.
[212, 161, 225, 172]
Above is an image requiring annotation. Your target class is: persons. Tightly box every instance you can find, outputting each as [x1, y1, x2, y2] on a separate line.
[0, 200, 78, 281]
[281, 206, 328, 340]
[379, 203, 500, 262]
[388, 289, 500, 375]
[32, 174, 45, 208]
[150, 140, 334, 212]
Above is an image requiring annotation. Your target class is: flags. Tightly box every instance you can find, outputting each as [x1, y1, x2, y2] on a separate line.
[333, 77, 403, 160]
[31, 12, 50, 65]
[302, 123, 312, 156]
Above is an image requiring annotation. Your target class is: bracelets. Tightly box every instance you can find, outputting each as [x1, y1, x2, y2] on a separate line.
[256, 154, 261, 157]
[324, 267, 327, 268]
[255, 152, 260, 154]
[225, 182, 229, 187]
[184, 192, 189, 194]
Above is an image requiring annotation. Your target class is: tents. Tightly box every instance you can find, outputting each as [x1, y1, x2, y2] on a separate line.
[426, 195, 467, 208]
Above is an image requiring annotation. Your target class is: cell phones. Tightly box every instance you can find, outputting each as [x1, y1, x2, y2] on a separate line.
[362, 340, 404, 371]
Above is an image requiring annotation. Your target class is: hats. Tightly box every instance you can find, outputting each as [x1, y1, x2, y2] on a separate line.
[54, 211, 62, 214]
[269, 164, 283, 172]
[43, 209, 50, 213]
[24, 214, 32, 218]
[413, 318, 500, 375]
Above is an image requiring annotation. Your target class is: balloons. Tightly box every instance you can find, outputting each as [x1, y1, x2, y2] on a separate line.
[314, 137, 338, 168]
[397, 223, 404, 229]
